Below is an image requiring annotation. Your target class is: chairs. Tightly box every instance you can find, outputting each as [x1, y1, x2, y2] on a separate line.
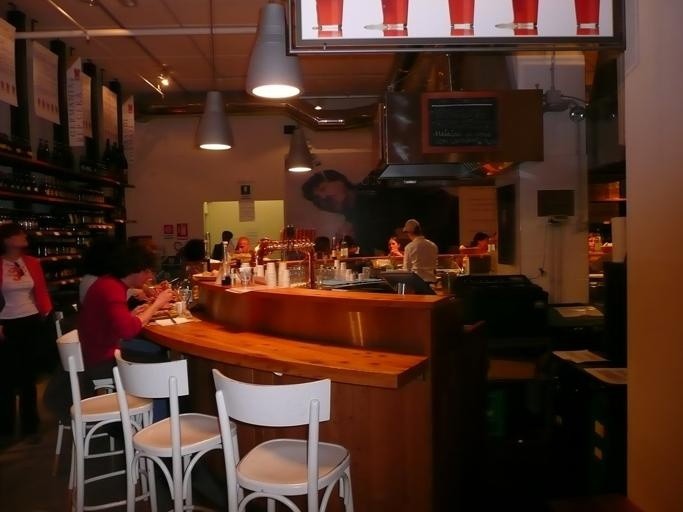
[52, 319, 115, 479]
[213, 369, 355, 512]
[55, 329, 158, 512]
[113, 349, 245, 512]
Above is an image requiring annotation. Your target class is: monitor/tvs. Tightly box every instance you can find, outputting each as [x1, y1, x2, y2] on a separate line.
[381, 272, 437, 295]
[537, 190, 575, 216]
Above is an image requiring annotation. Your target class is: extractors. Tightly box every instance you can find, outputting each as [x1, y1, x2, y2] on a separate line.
[356, 160, 498, 193]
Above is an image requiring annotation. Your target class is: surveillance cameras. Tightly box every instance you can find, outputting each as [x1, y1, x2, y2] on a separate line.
[567, 101, 586, 121]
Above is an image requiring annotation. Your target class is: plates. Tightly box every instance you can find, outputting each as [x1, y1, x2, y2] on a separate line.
[192, 273, 215, 278]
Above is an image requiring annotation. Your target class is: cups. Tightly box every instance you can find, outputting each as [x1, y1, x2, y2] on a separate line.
[332, 259, 354, 283]
[358, 274, 365, 281]
[607, 181, 620, 199]
[361, 265, 371, 280]
[228, 260, 289, 289]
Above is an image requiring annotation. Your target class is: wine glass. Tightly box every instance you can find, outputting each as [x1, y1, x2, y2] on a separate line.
[288, 264, 333, 290]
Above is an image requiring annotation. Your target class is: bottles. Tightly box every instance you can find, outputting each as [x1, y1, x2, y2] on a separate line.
[462, 254, 469, 274]
[330, 237, 337, 258]
[486, 242, 497, 275]
[219, 241, 231, 286]
[0, 132, 128, 255]
[42, 261, 83, 282]
[340, 241, 361, 259]
[593, 233, 601, 252]
[279, 223, 314, 261]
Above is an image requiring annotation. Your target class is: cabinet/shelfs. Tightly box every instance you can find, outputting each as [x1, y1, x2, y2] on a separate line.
[0, 146, 137, 324]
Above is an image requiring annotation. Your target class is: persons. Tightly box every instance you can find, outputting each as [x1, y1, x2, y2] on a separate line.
[313, 237, 331, 258]
[588, 233, 602, 256]
[387, 237, 405, 257]
[339, 236, 368, 257]
[74, 246, 175, 490]
[303, 169, 458, 253]
[403, 219, 439, 292]
[235, 237, 256, 267]
[212, 231, 233, 260]
[471, 233, 496, 275]
[0, 225, 52, 452]
[78, 243, 144, 309]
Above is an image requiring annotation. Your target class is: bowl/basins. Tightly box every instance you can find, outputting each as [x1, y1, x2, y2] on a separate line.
[599, 245, 611, 253]
[147, 286, 190, 304]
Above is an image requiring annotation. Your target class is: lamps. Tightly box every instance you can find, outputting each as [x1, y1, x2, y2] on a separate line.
[244, 0, 302, 101]
[195, 2, 234, 150]
[284, 109, 314, 172]
